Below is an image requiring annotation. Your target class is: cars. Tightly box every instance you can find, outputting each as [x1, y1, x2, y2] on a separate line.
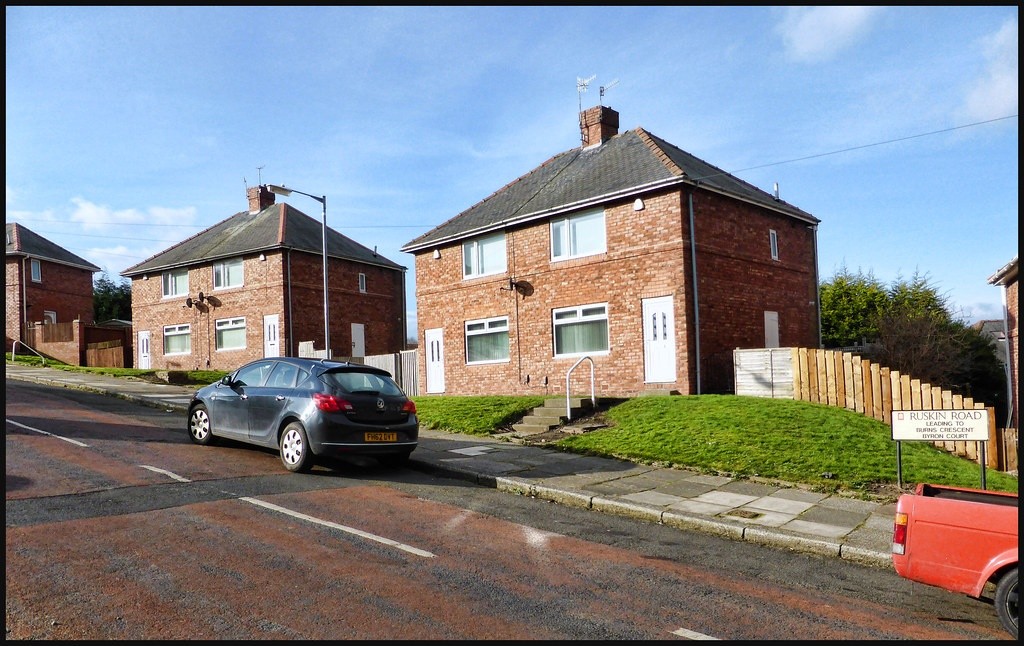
[187, 356, 419, 473]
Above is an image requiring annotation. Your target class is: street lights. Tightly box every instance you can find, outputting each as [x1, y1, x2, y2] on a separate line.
[266, 184, 330, 359]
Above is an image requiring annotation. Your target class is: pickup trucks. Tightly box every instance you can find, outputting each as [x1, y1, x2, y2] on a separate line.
[892, 482, 1018, 639]
